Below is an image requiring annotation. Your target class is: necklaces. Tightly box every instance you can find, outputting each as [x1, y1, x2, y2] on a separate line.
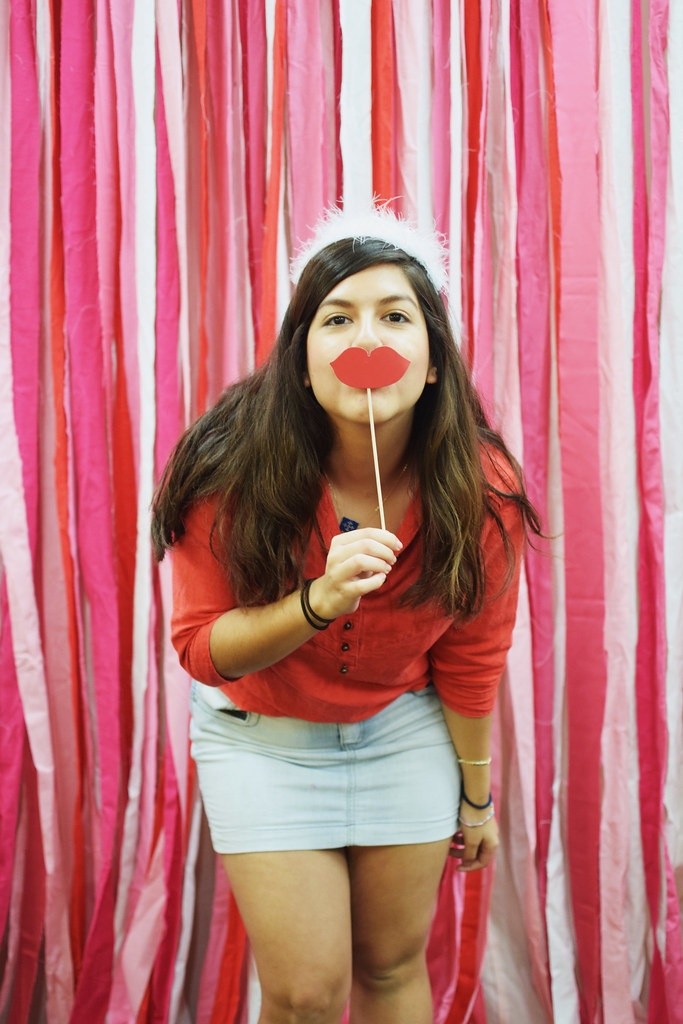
[328, 463, 408, 533]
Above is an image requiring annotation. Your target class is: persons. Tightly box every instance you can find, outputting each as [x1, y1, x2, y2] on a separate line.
[149, 204, 546, 1024]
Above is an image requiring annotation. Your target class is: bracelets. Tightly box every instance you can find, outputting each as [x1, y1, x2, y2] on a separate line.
[461, 781, 492, 809]
[459, 803, 495, 827]
[458, 758, 492, 766]
[300, 579, 329, 630]
[305, 578, 336, 623]
